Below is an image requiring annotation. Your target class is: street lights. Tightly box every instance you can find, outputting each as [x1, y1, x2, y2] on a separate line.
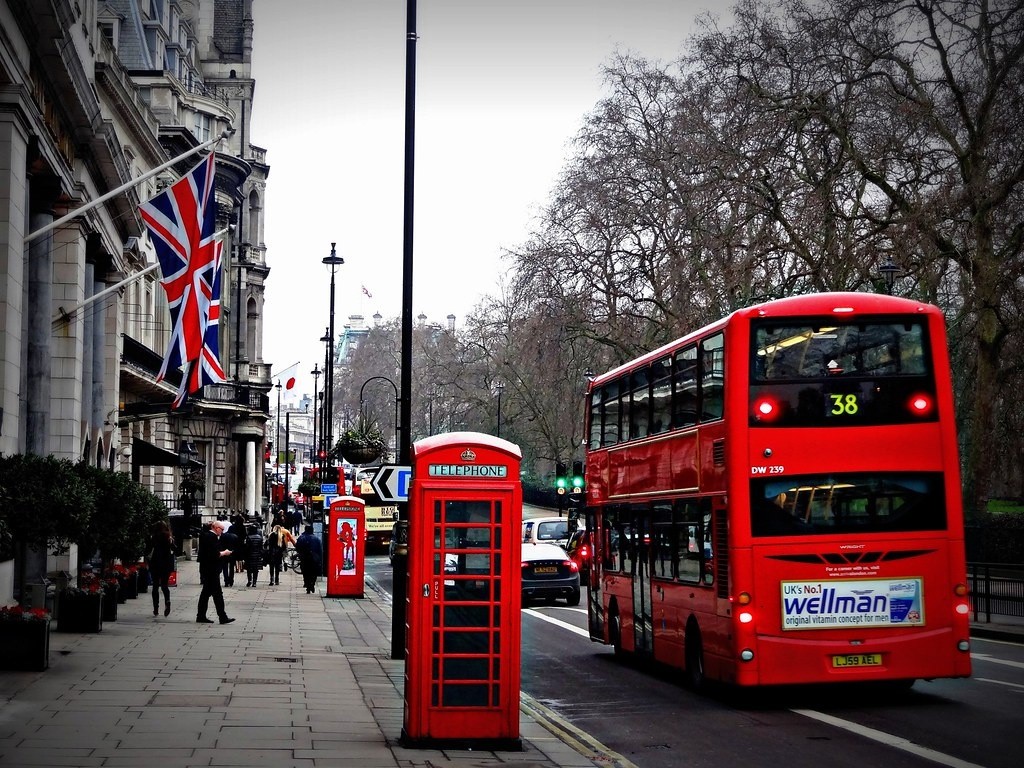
[311, 242, 346, 485]
[274, 380, 283, 482]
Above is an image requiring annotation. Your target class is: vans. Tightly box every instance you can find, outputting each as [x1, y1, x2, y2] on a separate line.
[521, 517, 583, 549]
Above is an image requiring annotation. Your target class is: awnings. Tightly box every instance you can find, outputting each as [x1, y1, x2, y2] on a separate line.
[133, 434, 208, 469]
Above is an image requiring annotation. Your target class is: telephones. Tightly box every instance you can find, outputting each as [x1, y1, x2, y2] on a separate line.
[434, 527, 459, 561]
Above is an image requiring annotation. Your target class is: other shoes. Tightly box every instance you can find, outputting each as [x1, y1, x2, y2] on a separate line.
[269, 582, 274, 586]
[153, 606, 159, 616]
[246, 582, 251, 587]
[219, 615, 236, 624]
[196, 617, 214, 623]
[252, 582, 256, 587]
[164, 601, 171, 616]
[225, 582, 233, 587]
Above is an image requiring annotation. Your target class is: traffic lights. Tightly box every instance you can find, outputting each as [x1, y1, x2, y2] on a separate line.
[556, 463, 566, 488]
[572, 461, 582, 487]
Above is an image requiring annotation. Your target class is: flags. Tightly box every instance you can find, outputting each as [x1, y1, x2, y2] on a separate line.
[140, 149, 231, 409]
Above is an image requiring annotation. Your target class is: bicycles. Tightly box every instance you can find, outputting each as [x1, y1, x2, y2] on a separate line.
[282, 548, 302, 574]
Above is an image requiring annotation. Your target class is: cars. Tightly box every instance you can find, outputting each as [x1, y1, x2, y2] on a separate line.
[264, 462, 303, 510]
[559, 529, 588, 586]
[520, 543, 581, 607]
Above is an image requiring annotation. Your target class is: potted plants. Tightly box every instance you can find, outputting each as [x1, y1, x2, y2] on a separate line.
[298, 480, 321, 497]
[331, 409, 389, 465]
[0, 450, 171, 671]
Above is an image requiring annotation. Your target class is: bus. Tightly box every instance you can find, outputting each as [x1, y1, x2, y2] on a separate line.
[582, 291, 972, 699]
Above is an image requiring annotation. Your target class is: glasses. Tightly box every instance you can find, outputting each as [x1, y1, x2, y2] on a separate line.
[217, 527, 224, 531]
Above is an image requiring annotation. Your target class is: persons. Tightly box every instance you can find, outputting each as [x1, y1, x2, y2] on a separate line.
[767, 351, 801, 379]
[297, 525, 322, 594]
[764, 480, 808, 524]
[668, 391, 719, 430]
[144, 521, 174, 617]
[852, 357, 871, 375]
[196, 520, 235, 624]
[197, 509, 303, 587]
